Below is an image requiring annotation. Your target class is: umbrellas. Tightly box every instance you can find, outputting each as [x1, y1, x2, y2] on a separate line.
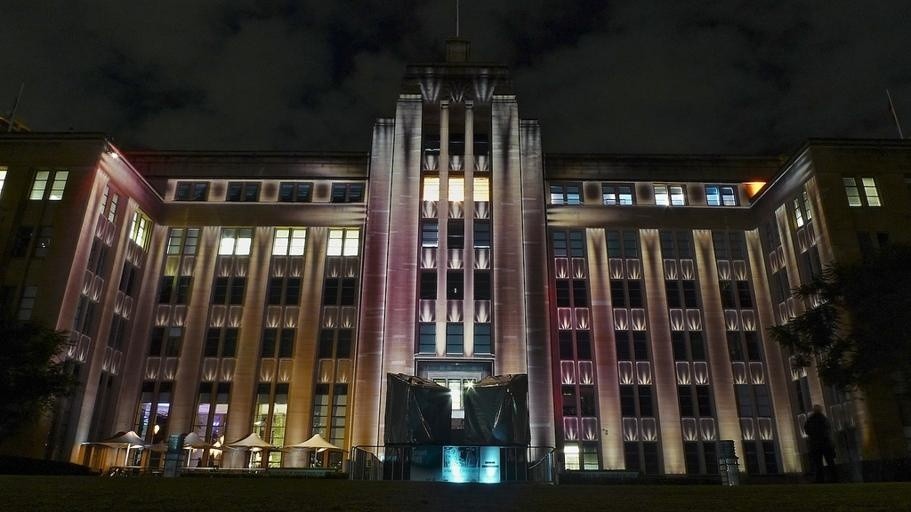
[282, 433, 343, 467]
[79, 429, 152, 465]
[81, 431, 126, 464]
[181, 430, 208, 467]
[227, 432, 274, 467]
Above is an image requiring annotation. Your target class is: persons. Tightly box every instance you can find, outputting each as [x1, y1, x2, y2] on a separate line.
[804, 403, 841, 484]
